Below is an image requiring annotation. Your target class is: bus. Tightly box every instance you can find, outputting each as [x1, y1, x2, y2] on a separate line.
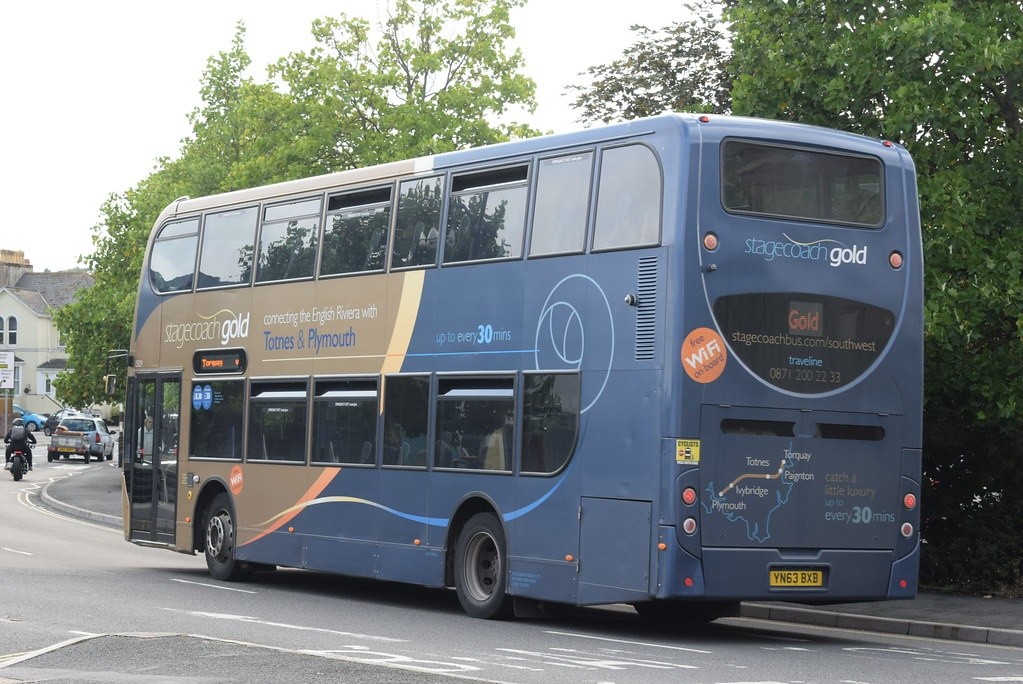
[106, 111, 925, 628]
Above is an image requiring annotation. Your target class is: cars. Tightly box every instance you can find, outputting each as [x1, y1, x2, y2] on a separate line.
[12, 404, 49, 432]
[43, 407, 94, 436]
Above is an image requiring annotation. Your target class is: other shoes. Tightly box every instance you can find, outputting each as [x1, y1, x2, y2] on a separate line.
[28, 467, 32, 470]
[4, 465, 9, 469]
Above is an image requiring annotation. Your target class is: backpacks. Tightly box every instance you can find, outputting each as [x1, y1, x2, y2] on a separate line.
[11, 425, 25, 441]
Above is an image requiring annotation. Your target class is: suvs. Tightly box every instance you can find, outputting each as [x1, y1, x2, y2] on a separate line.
[54, 413, 116, 462]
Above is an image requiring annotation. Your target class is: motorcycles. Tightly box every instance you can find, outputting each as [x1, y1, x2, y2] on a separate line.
[3, 440, 37, 481]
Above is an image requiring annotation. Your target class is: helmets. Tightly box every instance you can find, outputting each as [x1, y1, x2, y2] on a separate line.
[13, 418, 23, 426]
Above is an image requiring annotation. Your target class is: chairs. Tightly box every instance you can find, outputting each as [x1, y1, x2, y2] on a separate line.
[222, 428, 570, 473]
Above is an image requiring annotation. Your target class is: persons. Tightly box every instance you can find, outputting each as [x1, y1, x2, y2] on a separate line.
[137, 418, 164, 463]
[4, 418, 38, 472]
